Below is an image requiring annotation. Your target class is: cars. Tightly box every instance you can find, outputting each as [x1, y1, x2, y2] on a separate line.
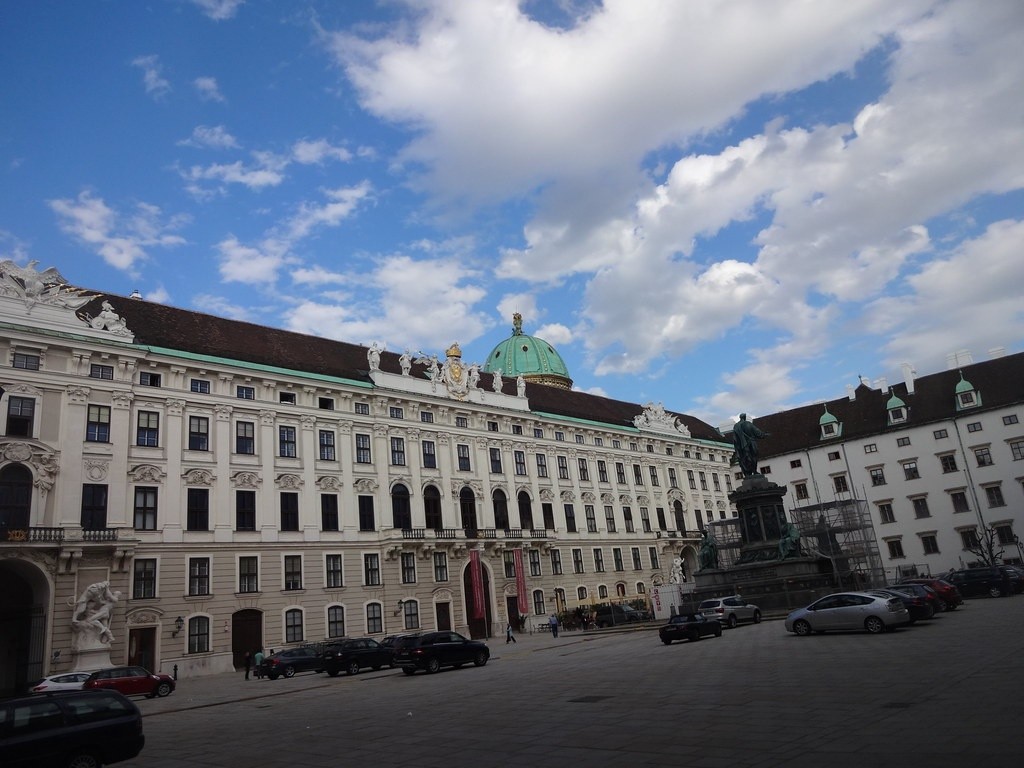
[83, 667, 175, 699]
[658, 613, 722, 645]
[324, 637, 380, 676]
[28, 672, 92, 694]
[253, 647, 323, 680]
[784, 578, 964, 635]
[594, 605, 651, 628]
[376, 631, 491, 673]
[697, 596, 763, 628]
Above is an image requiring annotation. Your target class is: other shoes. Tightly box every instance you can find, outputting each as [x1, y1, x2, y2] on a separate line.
[507, 643, 510, 644]
[514, 641, 516, 643]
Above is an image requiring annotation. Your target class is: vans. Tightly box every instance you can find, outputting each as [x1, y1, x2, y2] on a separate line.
[945, 565, 1021, 597]
[0, 688, 146, 768]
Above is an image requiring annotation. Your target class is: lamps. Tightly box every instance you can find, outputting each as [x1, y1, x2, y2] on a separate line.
[172, 616, 184, 638]
[550, 587, 558, 601]
[394, 599, 404, 615]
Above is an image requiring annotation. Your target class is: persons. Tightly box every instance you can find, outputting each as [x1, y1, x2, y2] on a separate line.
[467, 362, 483, 384]
[428, 354, 440, 380]
[778, 522, 800, 557]
[367, 341, 385, 370]
[244, 649, 276, 681]
[698, 530, 718, 569]
[398, 348, 413, 374]
[33, 454, 57, 491]
[517, 373, 526, 395]
[493, 368, 504, 390]
[88, 583, 122, 644]
[733, 413, 771, 476]
[671, 557, 685, 584]
[506, 623, 517, 644]
[581, 613, 588, 630]
[549, 614, 558, 638]
